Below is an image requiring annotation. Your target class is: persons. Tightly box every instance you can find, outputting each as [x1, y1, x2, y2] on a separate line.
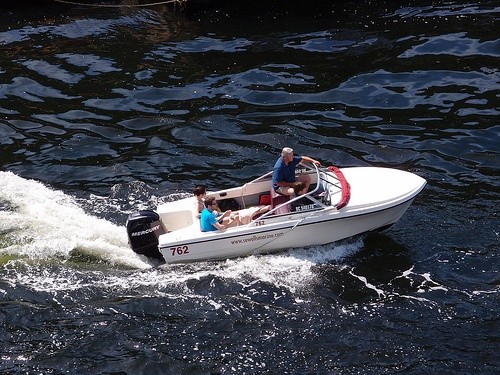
[193, 184, 207, 215]
[200, 196, 240, 231]
[272, 147, 321, 207]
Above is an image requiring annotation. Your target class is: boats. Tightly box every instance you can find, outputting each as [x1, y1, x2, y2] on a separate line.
[126, 159, 427, 264]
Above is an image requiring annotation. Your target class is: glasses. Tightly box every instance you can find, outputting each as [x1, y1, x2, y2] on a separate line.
[212, 203, 217, 205]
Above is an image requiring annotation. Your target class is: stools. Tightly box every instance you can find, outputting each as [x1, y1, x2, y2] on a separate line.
[270, 188, 290, 214]
